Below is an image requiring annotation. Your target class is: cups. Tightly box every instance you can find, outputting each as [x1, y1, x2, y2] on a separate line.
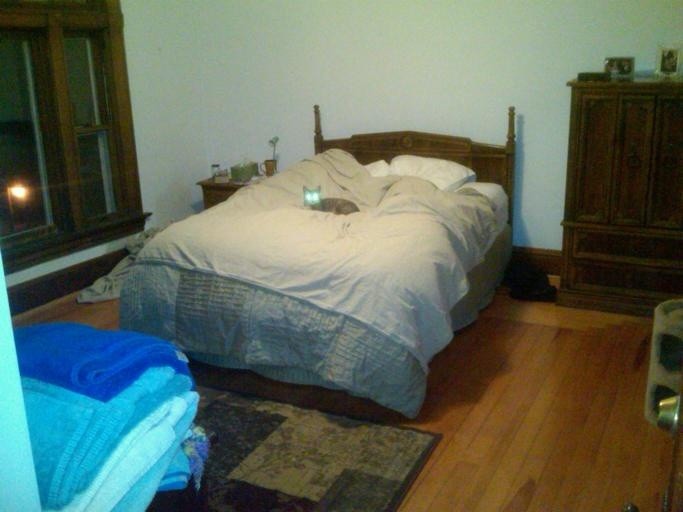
[260, 160, 276, 178]
[213, 176, 229, 183]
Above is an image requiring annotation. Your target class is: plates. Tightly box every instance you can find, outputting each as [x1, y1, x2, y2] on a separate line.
[577, 73, 608, 83]
[229, 179, 249, 186]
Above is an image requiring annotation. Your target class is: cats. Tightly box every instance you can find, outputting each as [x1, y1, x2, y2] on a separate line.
[302, 185, 360, 216]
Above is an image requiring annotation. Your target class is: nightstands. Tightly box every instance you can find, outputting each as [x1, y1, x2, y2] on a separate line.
[195, 171, 269, 210]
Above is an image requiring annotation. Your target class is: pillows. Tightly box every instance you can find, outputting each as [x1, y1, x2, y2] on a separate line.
[388, 153, 477, 192]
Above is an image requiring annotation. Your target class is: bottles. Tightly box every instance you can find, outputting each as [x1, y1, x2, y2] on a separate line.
[211, 165, 219, 178]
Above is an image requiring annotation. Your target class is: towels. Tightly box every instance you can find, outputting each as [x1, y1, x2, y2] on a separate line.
[13, 320, 200, 509]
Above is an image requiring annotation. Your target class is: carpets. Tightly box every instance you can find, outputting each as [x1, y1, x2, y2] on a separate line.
[151, 385, 444, 512]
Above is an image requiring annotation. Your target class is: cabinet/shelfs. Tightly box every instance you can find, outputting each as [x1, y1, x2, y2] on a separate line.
[555, 73, 682, 316]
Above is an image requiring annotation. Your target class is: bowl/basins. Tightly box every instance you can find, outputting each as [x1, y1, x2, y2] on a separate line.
[230, 163, 258, 180]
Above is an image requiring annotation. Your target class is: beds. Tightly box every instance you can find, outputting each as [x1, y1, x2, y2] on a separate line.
[131, 104, 518, 402]
